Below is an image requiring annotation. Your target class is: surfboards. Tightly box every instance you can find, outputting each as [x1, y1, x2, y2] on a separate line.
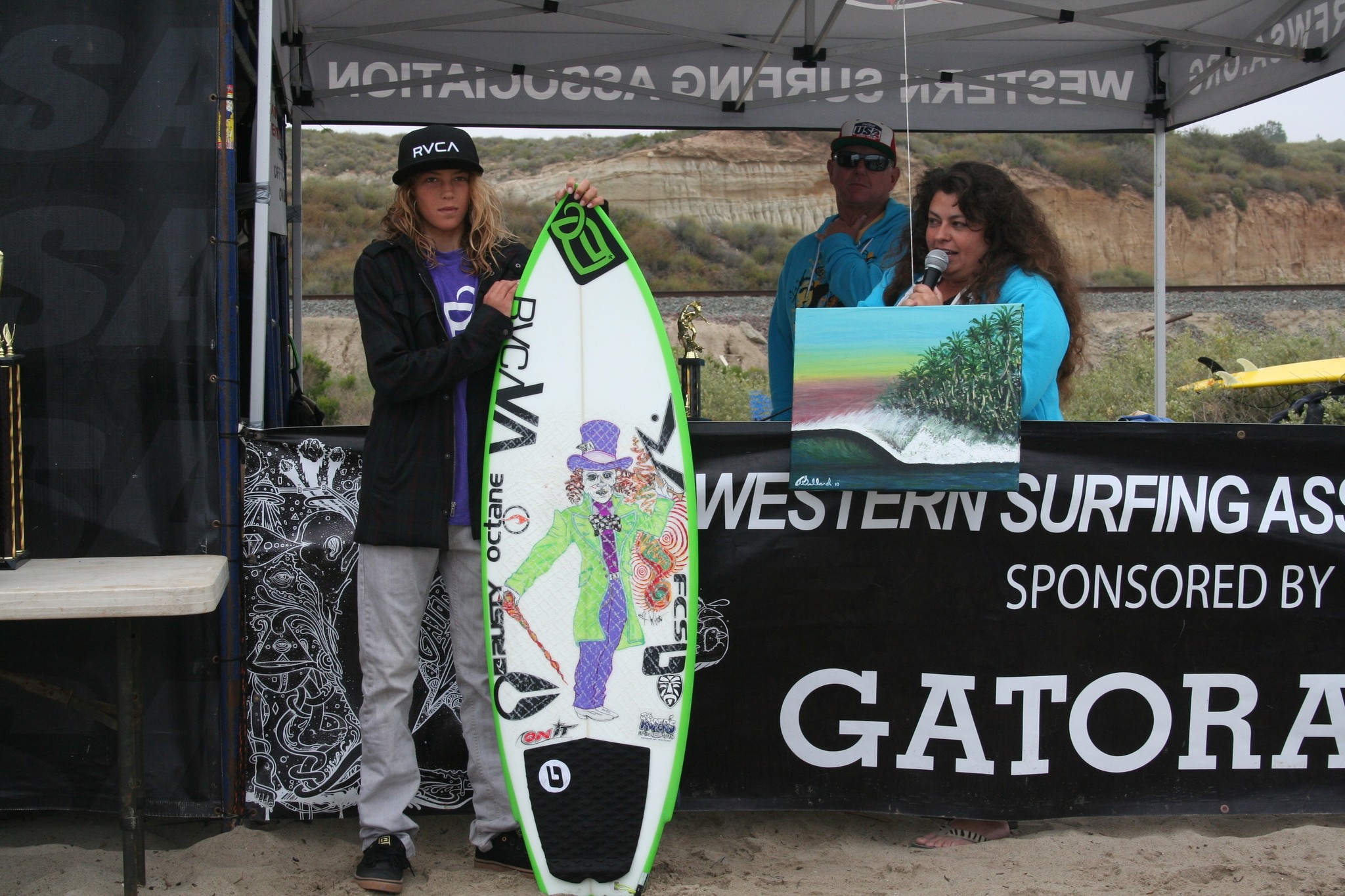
[479, 177, 698, 896]
[1175, 357, 1345, 395]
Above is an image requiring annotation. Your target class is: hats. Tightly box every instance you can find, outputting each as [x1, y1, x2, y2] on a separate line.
[830, 118, 896, 167]
[392, 125, 484, 187]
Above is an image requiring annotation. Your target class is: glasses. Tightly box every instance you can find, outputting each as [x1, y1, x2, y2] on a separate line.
[832, 151, 895, 171]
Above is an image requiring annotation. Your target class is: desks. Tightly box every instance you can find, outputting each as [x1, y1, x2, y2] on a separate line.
[0, 554, 230, 896]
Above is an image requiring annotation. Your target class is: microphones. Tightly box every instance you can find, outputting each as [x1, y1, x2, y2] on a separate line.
[922, 250, 949, 291]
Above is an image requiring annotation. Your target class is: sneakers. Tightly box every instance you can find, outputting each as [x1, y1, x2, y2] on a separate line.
[353, 834, 415, 892]
[474, 831, 535, 877]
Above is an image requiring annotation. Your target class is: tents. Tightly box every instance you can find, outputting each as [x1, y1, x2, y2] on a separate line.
[250, 0, 1344, 425]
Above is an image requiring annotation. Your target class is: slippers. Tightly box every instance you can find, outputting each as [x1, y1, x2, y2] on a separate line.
[912, 823, 985, 848]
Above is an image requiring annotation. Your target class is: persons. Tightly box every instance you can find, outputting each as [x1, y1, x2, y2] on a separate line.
[768, 119, 915, 419]
[350, 124, 610, 891]
[855, 159, 1082, 421]
